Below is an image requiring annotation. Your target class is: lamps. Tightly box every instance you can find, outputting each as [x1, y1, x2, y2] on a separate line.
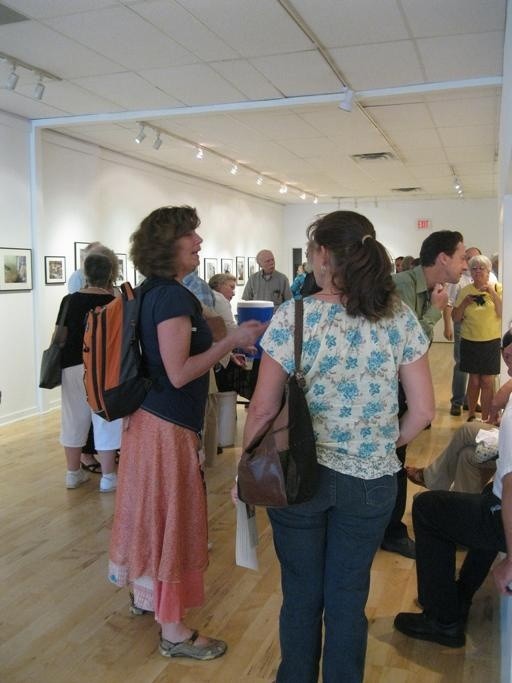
[337, 85, 358, 113]
[5, 64, 22, 91]
[153, 130, 164, 151]
[135, 122, 148, 145]
[31, 77, 45, 102]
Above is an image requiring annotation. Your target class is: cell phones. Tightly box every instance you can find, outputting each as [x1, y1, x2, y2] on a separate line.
[473, 294, 486, 297]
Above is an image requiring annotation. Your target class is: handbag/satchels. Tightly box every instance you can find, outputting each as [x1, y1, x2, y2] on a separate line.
[237, 298, 319, 509]
[39, 293, 72, 390]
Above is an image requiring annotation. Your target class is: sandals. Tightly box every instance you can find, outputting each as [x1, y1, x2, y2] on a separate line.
[79, 456, 102, 474]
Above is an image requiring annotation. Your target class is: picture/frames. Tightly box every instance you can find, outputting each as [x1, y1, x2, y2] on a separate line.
[0, 242, 261, 293]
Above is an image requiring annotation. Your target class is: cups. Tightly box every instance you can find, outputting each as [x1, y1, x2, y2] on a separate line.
[245, 356, 254, 370]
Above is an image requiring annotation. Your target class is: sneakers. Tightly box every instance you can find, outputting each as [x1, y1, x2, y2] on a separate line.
[464, 399, 483, 413]
[380, 532, 418, 561]
[158, 630, 228, 661]
[99, 475, 119, 493]
[65, 469, 89, 490]
[450, 402, 462, 416]
[405, 464, 428, 488]
[128, 595, 147, 615]
[395, 609, 471, 648]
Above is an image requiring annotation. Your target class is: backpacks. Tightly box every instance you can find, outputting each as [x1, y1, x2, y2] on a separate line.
[80, 282, 204, 422]
